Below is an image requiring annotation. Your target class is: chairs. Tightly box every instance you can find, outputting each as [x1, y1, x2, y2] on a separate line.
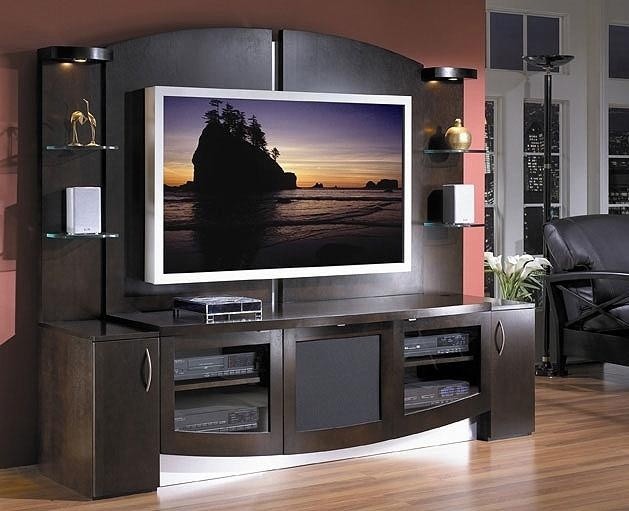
[537, 214, 629, 375]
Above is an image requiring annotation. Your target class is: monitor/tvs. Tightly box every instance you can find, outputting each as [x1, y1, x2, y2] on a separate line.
[145, 86, 414, 284]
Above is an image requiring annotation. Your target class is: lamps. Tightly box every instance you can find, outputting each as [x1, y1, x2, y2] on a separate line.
[523, 55, 576, 375]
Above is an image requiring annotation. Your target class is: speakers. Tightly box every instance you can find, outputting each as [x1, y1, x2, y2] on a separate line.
[442, 185, 476, 224]
[66, 188, 103, 237]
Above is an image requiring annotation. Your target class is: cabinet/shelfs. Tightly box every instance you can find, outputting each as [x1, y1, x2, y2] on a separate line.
[37, 325, 159, 500]
[36, 46, 120, 337]
[419, 66, 488, 227]
[478, 304, 535, 441]
[159, 303, 490, 490]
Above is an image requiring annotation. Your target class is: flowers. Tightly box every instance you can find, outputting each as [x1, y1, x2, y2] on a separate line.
[484, 251, 552, 301]
